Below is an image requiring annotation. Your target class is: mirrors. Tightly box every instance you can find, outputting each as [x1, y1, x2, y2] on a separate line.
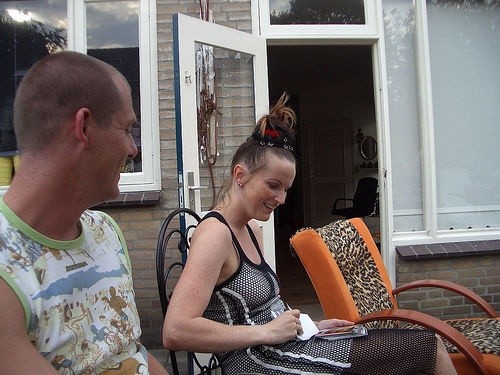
[360, 136, 377, 160]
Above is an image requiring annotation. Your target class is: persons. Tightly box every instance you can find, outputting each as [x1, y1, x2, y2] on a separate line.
[162, 92, 457, 375]
[0, 51, 169, 375]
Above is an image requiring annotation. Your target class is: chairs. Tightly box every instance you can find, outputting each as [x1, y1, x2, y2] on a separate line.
[331, 177, 379, 217]
[290, 217, 500, 375]
[156, 208, 222, 375]
